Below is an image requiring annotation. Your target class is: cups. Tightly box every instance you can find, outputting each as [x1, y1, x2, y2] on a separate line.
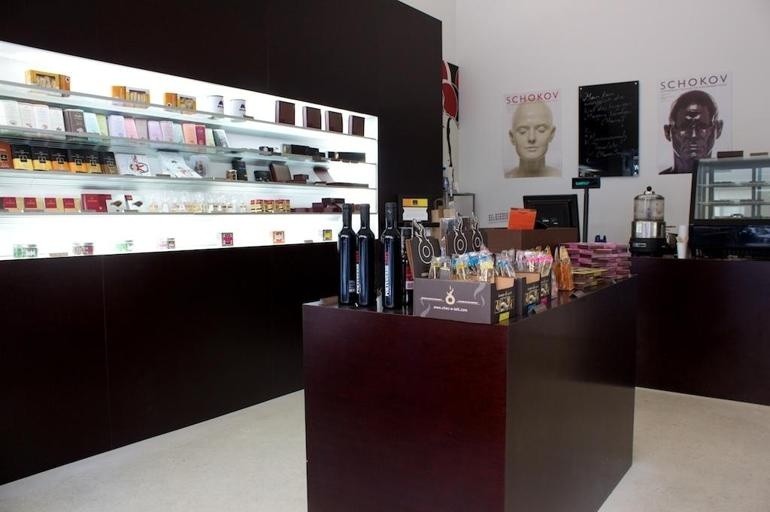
[675, 224, 692, 260]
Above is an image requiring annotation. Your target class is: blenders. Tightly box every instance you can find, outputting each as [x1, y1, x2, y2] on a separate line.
[627, 187, 669, 258]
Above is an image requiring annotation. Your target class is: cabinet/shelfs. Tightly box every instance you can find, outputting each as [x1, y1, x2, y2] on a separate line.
[0, 40, 378, 263]
[685, 157, 770, 262]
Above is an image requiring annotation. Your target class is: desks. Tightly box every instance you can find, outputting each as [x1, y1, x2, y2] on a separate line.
[299, 275, 638, 512]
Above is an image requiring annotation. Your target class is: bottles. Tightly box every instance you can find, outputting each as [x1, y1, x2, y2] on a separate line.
[354, 204, 379, 308]
[379, 201, 404, 310]
[338, 203, 358, 307]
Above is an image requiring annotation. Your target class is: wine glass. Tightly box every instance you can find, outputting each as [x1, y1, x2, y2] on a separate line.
[151, 189, 250, 213]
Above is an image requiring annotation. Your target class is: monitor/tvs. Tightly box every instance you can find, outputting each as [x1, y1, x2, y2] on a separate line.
[523, 194, 580, 242]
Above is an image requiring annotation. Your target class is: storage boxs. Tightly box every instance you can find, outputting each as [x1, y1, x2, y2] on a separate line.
[406, 228, 551, 326]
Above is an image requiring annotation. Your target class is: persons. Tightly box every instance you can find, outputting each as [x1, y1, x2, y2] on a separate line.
[659, 90, 723, 174]
[502, 97, 561, 178]
